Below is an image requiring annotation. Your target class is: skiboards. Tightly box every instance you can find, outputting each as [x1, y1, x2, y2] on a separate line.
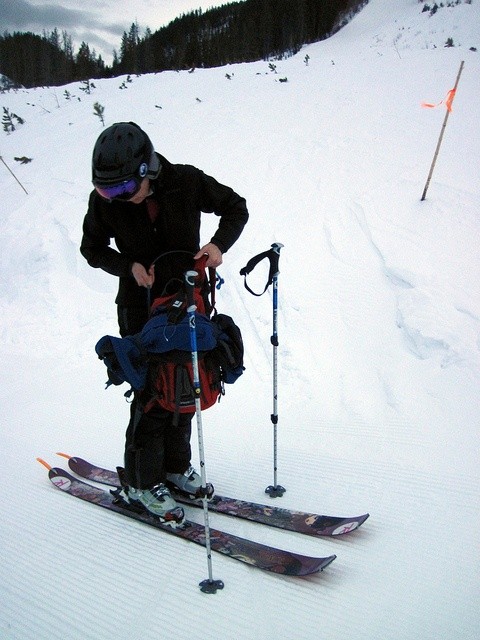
[48, 457, 369, 575]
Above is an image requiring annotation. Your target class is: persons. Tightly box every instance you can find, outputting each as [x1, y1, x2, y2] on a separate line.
[80, 121, 248, 520]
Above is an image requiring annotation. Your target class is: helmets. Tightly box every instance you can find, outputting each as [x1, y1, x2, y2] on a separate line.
[92, 122, 162, 203]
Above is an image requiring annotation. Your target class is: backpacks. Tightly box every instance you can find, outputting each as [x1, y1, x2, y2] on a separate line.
[142, 253, 225, 413]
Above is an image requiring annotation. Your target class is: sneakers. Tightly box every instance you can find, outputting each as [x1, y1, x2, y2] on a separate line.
[166, 467, 206, 495]
[127, 483, 178, 518]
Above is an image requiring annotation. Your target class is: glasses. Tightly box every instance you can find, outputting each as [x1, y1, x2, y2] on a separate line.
[93, 178, 141, 203]
